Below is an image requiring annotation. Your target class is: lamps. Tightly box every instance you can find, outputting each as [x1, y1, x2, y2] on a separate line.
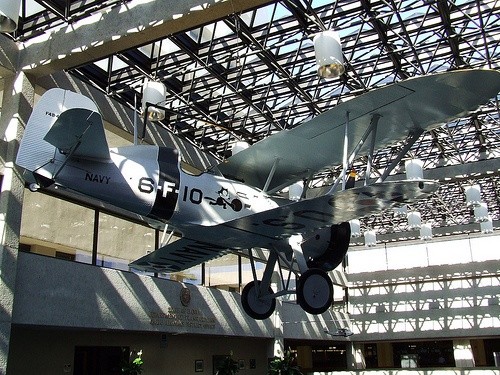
[141, 80, 165, 122]
[314, 30, 345, 78]
[464, 184, 494, 235]
[289, 180, 303, 202]
[348, 219, 381, 248]
[232, 142, 250, 156]
[394, 205, 433, 242]
[0, 0, 22, 33]
[405, 158, 424, 181]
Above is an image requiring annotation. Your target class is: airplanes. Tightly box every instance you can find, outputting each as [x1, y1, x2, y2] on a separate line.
[13, 69, 500, 321]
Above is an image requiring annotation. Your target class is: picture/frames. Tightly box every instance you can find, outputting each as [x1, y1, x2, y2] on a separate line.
[195, 359, 204, 372]
[238, 359, 246, 370]
